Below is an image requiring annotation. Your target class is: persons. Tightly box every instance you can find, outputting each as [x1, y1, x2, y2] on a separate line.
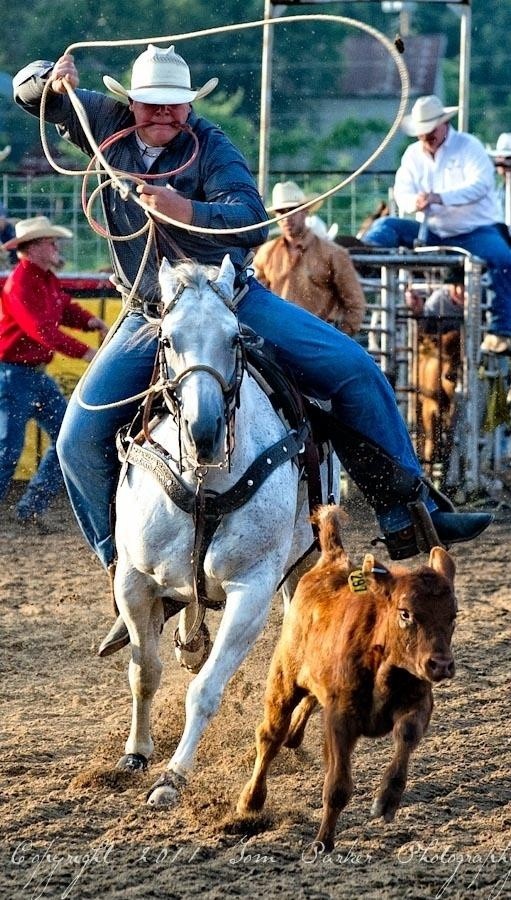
[487, 130, 511, 237]
[251, 177, 367, 337]
[0, 216, 110, 537]
[400, 260, 481, 334]
[356, 95, 511, 350]
[12, 43, 494, 658]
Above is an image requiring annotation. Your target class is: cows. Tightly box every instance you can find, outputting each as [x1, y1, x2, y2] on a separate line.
[238, 499, 459, 855]
[412, 334, 463, 484]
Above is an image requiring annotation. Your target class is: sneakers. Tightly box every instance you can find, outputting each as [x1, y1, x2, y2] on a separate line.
[479, 334, 510, 355]
[386, 510, 495, 561]
[334, 234, 371, 270]
[9, 509, 71, 534]
[98, 599, 186, 658]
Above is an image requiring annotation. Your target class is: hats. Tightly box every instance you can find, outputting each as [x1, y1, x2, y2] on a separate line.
[264, 180, 325, 214]
[443, 263, 487, 284]
[486, 133, 510, 159]
[102, 43, 220, 105]
[400, 95, 459, 138]
[1, 216, 74, 251]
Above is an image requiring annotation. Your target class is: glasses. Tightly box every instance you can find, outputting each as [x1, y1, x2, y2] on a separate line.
[33, 238, 56, 248]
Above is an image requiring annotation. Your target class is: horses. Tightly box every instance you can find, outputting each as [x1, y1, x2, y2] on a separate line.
[111, 252, 322, 816]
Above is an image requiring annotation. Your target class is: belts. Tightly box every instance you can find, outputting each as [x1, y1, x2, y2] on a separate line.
[9, 360, 47, 373]
